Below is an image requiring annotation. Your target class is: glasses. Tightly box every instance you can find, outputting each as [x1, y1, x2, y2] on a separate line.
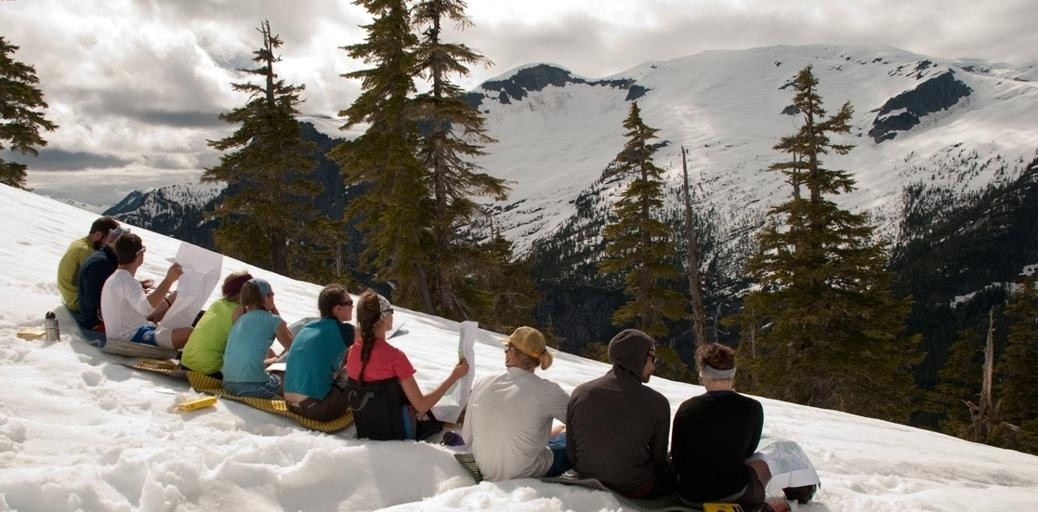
[382, 308, 394, 314]
[134, 246, 147, 256]
[338, 300, 353, 307]
[502, 343, 512, 352]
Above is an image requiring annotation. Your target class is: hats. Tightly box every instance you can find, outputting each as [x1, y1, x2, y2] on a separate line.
[501, 326, 553, 370]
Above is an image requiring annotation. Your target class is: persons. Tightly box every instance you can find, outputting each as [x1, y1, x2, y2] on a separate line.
[58, 216, 119, 328]
[670, 342, 820, 511]
[284, 285, 358, 423]
[565, 329, 676, 500]
[347, 292, 468, 440]
[220, 279, 296, 400]
[76, 228, 129, 331]
[462, 327, 574, 481]
[100, 233, 202, 349]
[181, 272, 256, 380]
